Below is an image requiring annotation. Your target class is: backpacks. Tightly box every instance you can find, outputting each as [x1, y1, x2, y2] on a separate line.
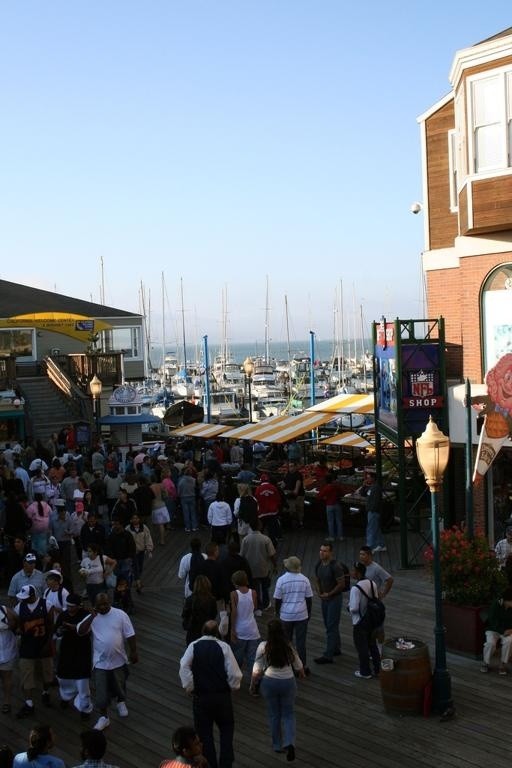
[334, 560, 350, 592]
[356, 582, 386, 630]
[237, 496, 258, 521]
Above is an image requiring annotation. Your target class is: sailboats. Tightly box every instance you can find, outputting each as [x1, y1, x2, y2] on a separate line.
[139, 267, 376, 435]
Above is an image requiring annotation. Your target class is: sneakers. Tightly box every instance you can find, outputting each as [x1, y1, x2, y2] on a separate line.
[286, 744, 296, 762]
[3, 703, 11, 713]
[313, 657, 333, 664]
[498, 665, 507, 676]
[185, 527, 200, 533]
[374, 545, 388, 552]
[324, 536, 344, 542]
[16, 702, 35, 719]
[355, 671, 372, 679]
[81, 711, 91, 721]
[116, 701, 128, 717]
[93, 715, 110, 731]
[41, 690, 51, 708]
[254, 601, 271, 617]
[480, 661, 488, 673]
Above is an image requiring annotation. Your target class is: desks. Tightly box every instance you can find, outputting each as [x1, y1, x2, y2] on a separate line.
[304, 482, 400, 531]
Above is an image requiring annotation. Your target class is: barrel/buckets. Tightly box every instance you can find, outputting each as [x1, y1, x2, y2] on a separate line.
[380, 638, 434, 714]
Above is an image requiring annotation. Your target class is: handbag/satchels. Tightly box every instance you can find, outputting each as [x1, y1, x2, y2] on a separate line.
[161, 488, 168, 500]
[248, 673, 262, 697]
[182, 615, 194, 631]
[218, 610, 229, 636]
[104, 570, 117, 589]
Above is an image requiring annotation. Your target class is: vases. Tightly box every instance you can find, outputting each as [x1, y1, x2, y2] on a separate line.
[437, 598, 503, 662]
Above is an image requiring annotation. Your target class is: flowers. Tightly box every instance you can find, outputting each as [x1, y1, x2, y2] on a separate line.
[422, 521, 512, 607]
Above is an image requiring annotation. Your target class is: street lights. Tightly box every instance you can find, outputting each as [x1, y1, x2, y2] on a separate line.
[88, 373, 102, 441]
[414, 411, 452, 716]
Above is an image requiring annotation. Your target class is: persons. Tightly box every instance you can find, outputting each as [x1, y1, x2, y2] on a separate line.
[1, 424, 512, 768]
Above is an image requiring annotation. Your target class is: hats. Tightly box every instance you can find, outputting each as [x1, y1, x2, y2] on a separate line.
[260, 474, 270, 481]
[25, 553, 37, 565]
[43, 570, 63, 584]
[354, 561, 366, 574]
[54, 499, 65, 507]
[16, 584, 36, 599]
[153, 442, 161, 451]
[75, 502, 84, 512]
[282, 557, 302, 573]
